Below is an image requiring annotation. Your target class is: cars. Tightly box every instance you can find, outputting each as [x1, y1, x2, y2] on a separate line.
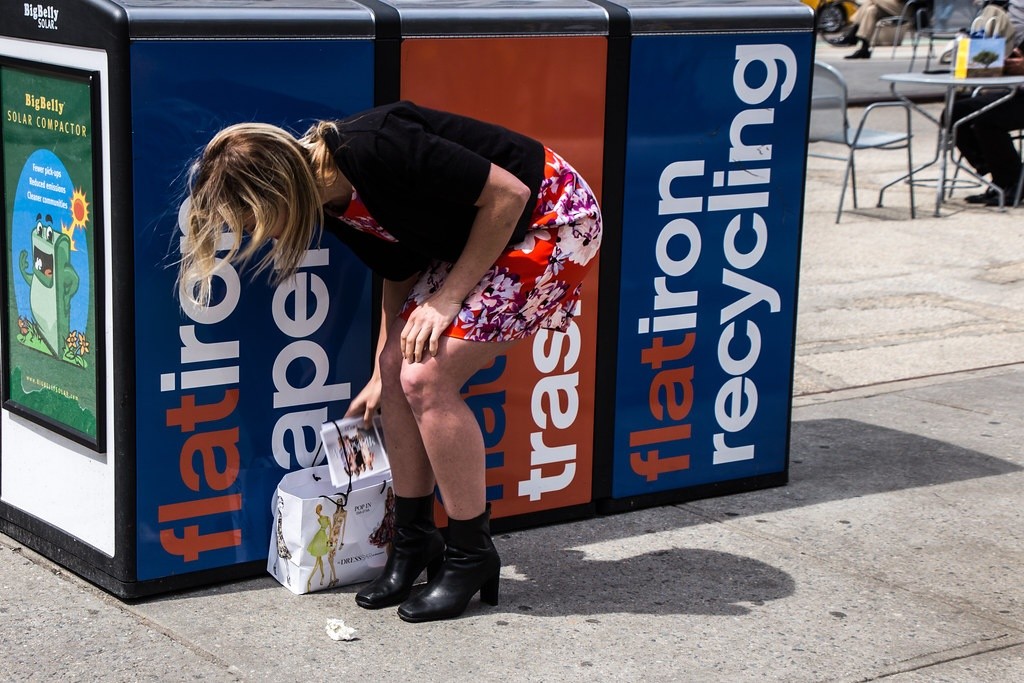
[801, 1, 860, 34]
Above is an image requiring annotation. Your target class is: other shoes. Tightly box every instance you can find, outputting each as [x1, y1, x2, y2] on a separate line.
[836, 34, 859, 45]
[845, 49, 871, 59]
[964, 164, 1023, 206]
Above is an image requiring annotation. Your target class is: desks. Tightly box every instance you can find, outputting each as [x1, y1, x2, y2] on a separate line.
[877, 71, 1024, 219]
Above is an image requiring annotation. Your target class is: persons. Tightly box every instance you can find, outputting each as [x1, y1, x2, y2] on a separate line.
[830, 0, 935, 59]
[185, 99, 603, 622]
[941, 0, 1024, 206]
[338, 432, 375, 477]
[272, 496, 293, 586]
[306, 498, 347, 592]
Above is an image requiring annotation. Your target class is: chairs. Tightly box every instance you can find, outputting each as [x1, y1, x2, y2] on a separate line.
[947, 127, 1024, 208]
[809, 59, 916, 224]
[869, 0, 934, 60]
[906, 0, 981, 73]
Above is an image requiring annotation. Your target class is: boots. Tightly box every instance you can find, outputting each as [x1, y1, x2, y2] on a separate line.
[354, 493, 445, 607]
[397, 500, 500, 622]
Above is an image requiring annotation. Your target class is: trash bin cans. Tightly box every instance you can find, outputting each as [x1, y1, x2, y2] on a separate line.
[1, 0, 815, 601]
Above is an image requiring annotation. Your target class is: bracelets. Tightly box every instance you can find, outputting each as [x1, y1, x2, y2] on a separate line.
[341, 543, 344, 546]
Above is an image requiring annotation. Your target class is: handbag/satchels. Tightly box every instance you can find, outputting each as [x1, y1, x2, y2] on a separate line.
[266, 421, 397, 594]
